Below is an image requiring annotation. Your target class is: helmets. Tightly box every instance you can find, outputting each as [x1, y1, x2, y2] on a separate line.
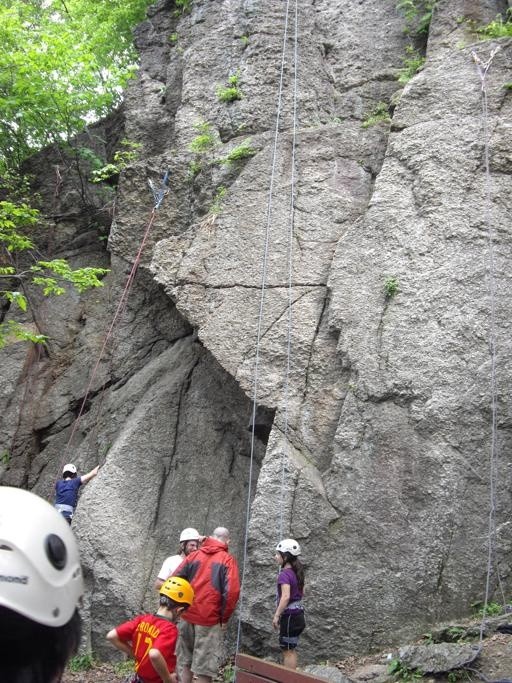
[276, 538, 302, 556]
[62, 463, 77, 474]
[160, 576, 194, 606]
[0, 486, 84, 629]
[180, 527, 202, 543]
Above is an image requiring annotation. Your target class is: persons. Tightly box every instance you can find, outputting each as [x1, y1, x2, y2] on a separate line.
[154, 528, 201, 591]
[0, 485, 85, 683]
[272, 539, 306, 671]
[53, 463, 99, 525]
[106, 576, 195, 683]
[172, 526, 241, 683]
